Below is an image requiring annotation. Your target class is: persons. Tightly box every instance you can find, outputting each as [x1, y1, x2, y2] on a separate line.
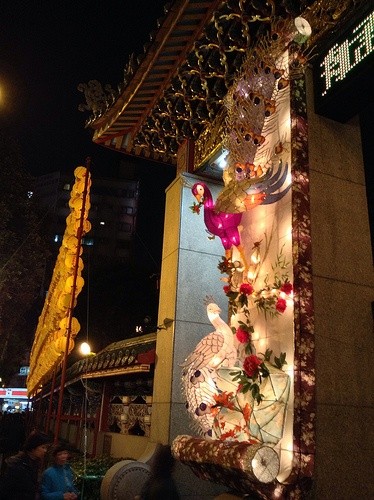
[38, 446, 78, 500]
[0, 431, 49, 500]
[0, 411, 26, 454]
[135, 451, 180, 500]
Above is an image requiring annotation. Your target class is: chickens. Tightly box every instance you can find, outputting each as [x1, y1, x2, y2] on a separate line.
[191, 158, 293, 272]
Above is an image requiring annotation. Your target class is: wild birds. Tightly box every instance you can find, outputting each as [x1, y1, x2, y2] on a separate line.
[180, 293, 245, 438]
